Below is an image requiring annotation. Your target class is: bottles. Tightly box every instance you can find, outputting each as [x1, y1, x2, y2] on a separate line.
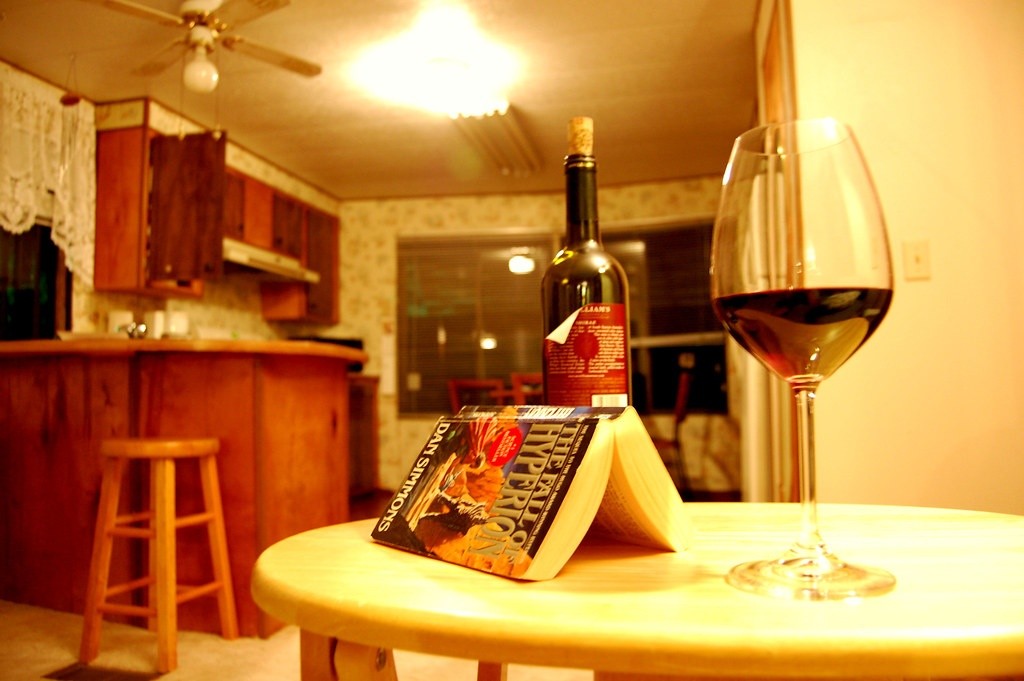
[542, 116, 631, 410]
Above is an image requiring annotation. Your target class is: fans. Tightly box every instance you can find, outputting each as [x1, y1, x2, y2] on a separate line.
[85, 0, 321, 79]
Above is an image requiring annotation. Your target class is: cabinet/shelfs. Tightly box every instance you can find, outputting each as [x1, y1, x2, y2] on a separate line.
[94, 95, 228, 303]
[350, 382, 381, 500]
[260, 179, 341, 326]
[226, 137, 302, 260]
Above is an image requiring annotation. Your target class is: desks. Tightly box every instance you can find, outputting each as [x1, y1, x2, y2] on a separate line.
[248, 503, 1024, 681]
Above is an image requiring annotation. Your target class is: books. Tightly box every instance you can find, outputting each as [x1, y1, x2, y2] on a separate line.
[370, 404, 694, 581]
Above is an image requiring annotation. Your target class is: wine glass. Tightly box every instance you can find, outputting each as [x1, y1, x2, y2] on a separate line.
[712, 117, 895, 603]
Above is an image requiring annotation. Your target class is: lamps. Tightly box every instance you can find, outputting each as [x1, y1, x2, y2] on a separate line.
[182, 45, 219, 94]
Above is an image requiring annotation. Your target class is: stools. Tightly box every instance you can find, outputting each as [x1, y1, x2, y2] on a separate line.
[77, 437, 239, 675]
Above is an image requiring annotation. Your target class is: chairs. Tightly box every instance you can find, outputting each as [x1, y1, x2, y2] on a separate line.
[445, 378, 504, 414]
[508, 372, 545, 406]
[653, 353, 695, 503]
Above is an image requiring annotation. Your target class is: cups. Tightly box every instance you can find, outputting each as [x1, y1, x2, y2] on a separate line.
[145, 312, 163, 340]
[165, 309, 189, 340]
[111, 310, 134, 337]
